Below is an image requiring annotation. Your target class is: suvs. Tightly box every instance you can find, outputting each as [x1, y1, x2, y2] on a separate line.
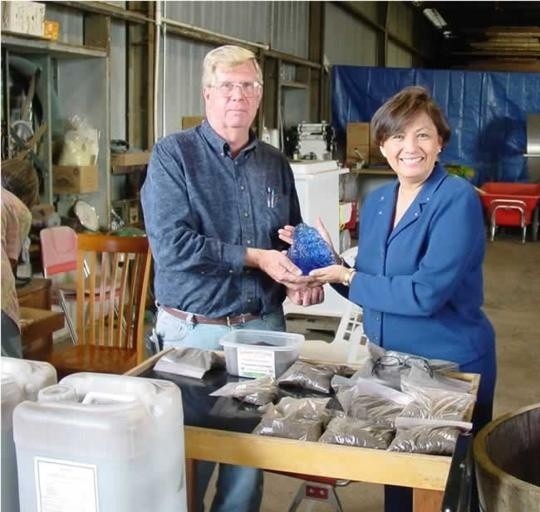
[1, 356, 57, 512]
[11, 373, 186, 512]
[473, 403, 540, 512]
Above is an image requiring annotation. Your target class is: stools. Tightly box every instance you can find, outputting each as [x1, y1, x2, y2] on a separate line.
[163, 306, 259, 325]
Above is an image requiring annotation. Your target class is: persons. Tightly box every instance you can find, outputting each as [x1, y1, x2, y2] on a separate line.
[277, 85, 496, 511]
[141, 43, 324, 512]
[1, 157, 39, 357]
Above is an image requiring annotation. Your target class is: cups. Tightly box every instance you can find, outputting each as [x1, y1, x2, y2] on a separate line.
[208, 81, 259, 99]
[373, 353, 433, 379]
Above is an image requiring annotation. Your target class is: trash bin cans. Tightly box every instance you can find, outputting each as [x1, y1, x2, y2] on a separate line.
[341, 267, 355, 287]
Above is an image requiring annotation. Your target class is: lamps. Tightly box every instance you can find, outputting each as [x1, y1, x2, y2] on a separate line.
[39, 224, 153, 379]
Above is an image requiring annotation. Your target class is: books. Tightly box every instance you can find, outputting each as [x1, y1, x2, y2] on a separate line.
[345, 122, 387, 164]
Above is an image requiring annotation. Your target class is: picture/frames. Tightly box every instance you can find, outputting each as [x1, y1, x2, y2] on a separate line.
[122, 347, 481, 511]
